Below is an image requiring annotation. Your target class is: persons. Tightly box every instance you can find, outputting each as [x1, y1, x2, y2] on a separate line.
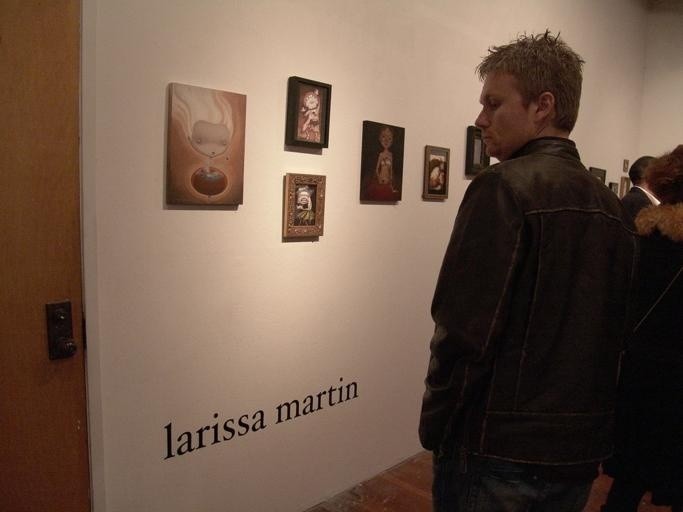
[171, 85, 233, 158]
[375, 126, 393, 185]
[301, 89, 321, 143]
[600, 148, 683, 510]
[296, 186, 313, 226]
[419, 30, 640, 512]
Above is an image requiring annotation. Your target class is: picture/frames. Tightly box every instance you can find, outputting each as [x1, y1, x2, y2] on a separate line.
[423, 125, 491, 199]
[280, 76, 332, 237]
